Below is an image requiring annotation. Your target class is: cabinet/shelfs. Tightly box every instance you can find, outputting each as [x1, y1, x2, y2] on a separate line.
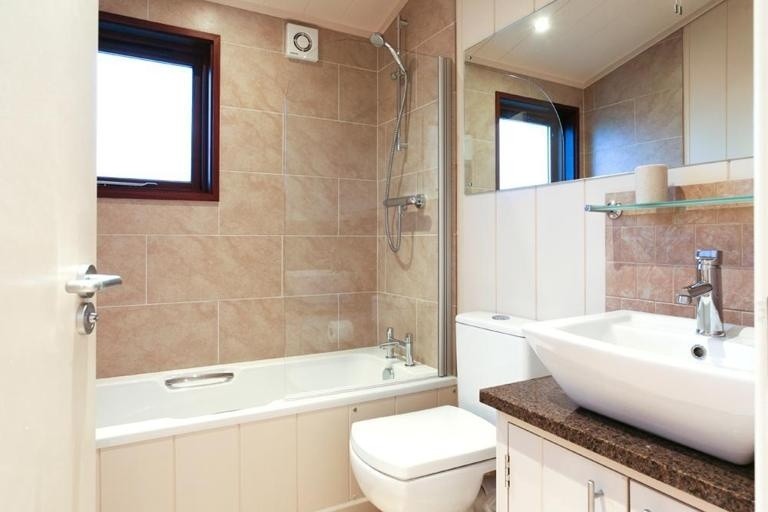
[495, 409, 736, 512]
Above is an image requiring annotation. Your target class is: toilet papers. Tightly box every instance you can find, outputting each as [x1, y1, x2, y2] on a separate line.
[634, 165, 670, 206]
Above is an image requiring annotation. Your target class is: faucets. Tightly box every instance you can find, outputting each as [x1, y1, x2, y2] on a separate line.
[677, 246, 727, 337]
[380, 328, 415, 366]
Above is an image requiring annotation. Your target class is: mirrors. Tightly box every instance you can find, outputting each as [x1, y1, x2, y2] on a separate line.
[464, 0, 753, 193]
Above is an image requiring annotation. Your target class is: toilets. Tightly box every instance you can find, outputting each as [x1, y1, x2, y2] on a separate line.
[346, 312, 549, 512]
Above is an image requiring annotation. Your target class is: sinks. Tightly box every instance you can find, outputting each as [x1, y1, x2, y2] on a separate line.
[518, 306, 754, 465]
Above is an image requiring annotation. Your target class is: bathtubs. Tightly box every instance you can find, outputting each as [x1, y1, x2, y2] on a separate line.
[93, 340, 455, 510]
